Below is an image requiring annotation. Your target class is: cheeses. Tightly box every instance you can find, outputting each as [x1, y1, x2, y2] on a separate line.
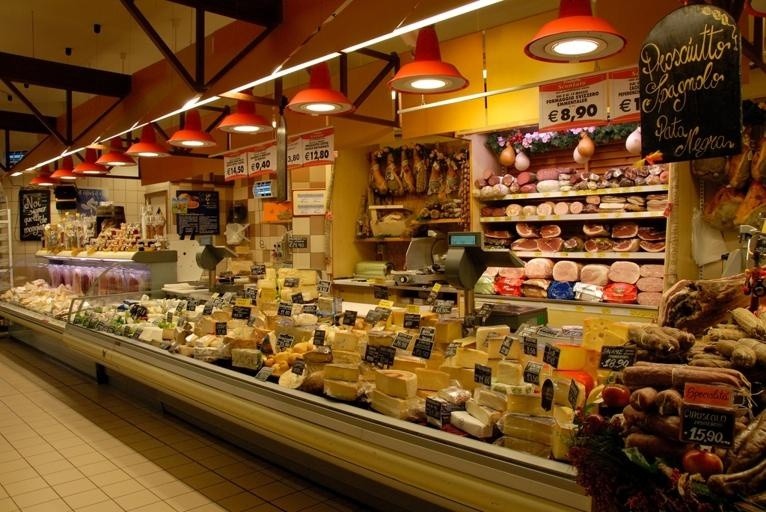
[135, 265, 653, 461]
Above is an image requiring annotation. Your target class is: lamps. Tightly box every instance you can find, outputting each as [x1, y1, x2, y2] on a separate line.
[126, 125, 171, 158]
[286, 61, 354, 115]
[522, 1, 628, 62]
[387, 25, 470, 95]
[29, 146, 111, 186]
[94, 136, 138, 167]
[219, 87, 274, 134]
[168, 110, 217, 148]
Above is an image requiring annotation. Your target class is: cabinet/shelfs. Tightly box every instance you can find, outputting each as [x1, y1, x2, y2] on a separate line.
[353, 203, 465, 243]
[0, 248, 177, 385]
[468, 115, 703, 310]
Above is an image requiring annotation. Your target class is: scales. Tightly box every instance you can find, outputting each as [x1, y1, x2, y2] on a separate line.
[444, 231, 548, 332]
[195, 234, 244, 293]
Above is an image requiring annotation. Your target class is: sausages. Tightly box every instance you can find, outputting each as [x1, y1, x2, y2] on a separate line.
[605, 308, 766, 476]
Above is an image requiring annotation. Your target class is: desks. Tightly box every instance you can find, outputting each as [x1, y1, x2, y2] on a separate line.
[333, 276, 473, 320]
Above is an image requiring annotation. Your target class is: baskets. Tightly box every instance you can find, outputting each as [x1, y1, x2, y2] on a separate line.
[656, 455, 766, 501]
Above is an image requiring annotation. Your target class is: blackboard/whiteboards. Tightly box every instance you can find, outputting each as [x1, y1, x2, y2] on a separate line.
[639, 4, 742, 165]
[19, 190, 50, 241]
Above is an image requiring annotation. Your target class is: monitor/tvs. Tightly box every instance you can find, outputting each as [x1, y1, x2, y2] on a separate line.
[9, 151, 29, 168]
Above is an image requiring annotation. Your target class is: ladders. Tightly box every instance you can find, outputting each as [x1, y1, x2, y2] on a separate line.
[0, 209, 13, 289]
